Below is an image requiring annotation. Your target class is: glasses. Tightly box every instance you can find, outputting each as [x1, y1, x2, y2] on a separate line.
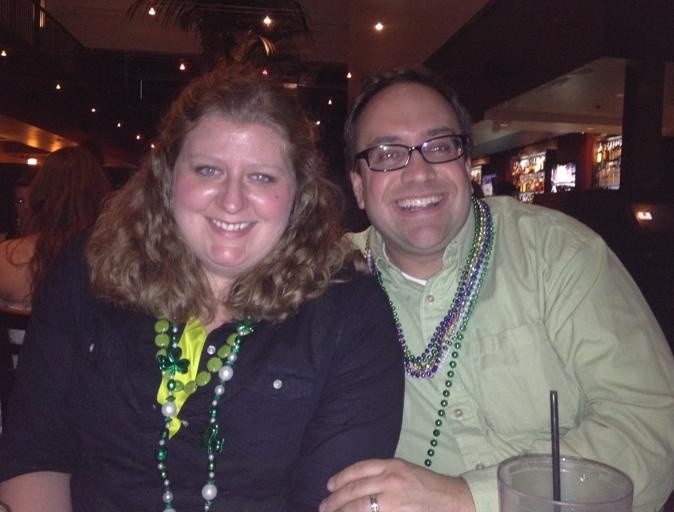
[355, 133, 470, 173]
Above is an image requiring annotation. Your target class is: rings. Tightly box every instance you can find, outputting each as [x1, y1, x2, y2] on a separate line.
[369, 493, 380, 512]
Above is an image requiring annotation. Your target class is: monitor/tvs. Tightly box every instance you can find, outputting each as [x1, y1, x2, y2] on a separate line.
[467, 137, 621, 205]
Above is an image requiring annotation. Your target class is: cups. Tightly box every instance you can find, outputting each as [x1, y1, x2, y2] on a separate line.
[497, 454, 633, 512]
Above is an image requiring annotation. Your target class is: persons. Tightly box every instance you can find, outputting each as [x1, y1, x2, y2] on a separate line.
[315, 67, 673, 512]
[6, 171, 43, 239]
[0, 57, 404, 512]
[0, 143, 115, 378]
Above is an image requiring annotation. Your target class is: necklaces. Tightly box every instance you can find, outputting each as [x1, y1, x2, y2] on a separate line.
[360, 196, 496, 480]
[155, 321, 262, 511]
[153, 316, 250, 397]
